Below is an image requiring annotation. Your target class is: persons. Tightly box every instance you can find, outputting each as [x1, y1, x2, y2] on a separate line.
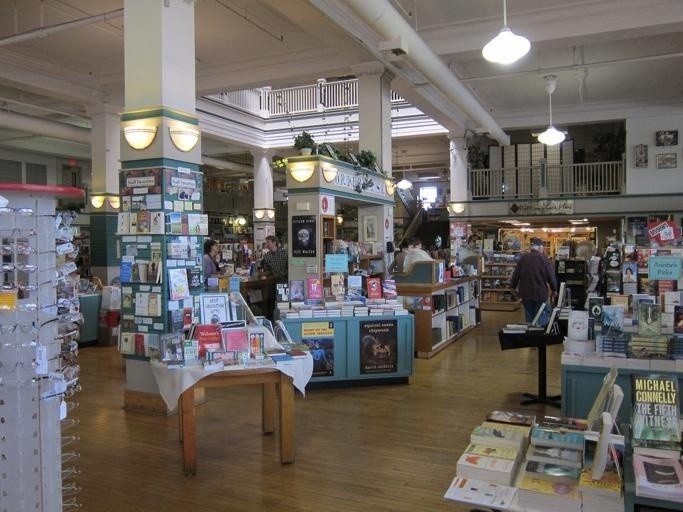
[202, 239, 228, 281]
[259, 235, 288, 324]
[509, 236, 557, 330]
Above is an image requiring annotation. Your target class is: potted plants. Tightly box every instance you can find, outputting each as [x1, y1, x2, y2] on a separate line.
[294, 131, 317, 156]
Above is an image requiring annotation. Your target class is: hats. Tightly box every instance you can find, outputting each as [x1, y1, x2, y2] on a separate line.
[530, 238, 543, 246]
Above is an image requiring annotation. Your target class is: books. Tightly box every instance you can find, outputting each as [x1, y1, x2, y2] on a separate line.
[556, 283, 564, 308]
[209, 273, 408, 366]
[441, 363, 683, 512]
[431, 282, 480, 348]
[528, 326, 543, 331]
[505, 322, 527, 330]
[530, 303, 545, 326]
[115, 194, 209, 365]
[564, 238, 682, 362]
[545, 307, 560, 334]
[502, 328, 526, 333]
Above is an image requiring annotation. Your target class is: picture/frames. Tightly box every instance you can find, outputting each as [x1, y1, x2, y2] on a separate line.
[654, 130, 678, 168]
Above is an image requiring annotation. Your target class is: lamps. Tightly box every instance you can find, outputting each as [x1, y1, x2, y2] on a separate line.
[452, 203, 464, 214]
[288, 162, 340, 183]
[91, 197, 121, 210]
[396, 164, 413, 190]
[254, 210, 275, 219]
[122, 124, 201, 152]
[480, 0, 568, 146]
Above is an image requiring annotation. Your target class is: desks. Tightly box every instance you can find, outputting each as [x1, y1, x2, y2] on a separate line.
[149, 350, 312, 478]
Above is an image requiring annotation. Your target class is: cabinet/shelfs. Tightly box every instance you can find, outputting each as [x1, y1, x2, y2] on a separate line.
[271, 308, 415, 389]
[121, 279, 164, 318]
[560, 362, 683, 425]
[320, 214, 336, 280]
[240, 278, 276, 320]
[341, 254, 523, 360]
[78, 294, 102, 345]
